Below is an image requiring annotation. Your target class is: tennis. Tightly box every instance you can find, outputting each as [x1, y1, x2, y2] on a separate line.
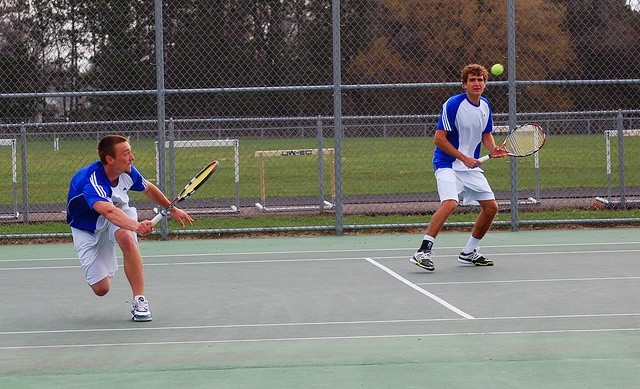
[491, 64, 504, 76]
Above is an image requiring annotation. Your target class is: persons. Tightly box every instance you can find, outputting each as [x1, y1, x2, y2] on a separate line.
[410, 64, 512, 271]
[66, 135, 194, 322]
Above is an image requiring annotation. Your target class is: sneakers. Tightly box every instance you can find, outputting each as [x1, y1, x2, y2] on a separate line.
[458, 248, 494, 267]
[125, 298, 153, 322]
[410, 250, 435, 271]
